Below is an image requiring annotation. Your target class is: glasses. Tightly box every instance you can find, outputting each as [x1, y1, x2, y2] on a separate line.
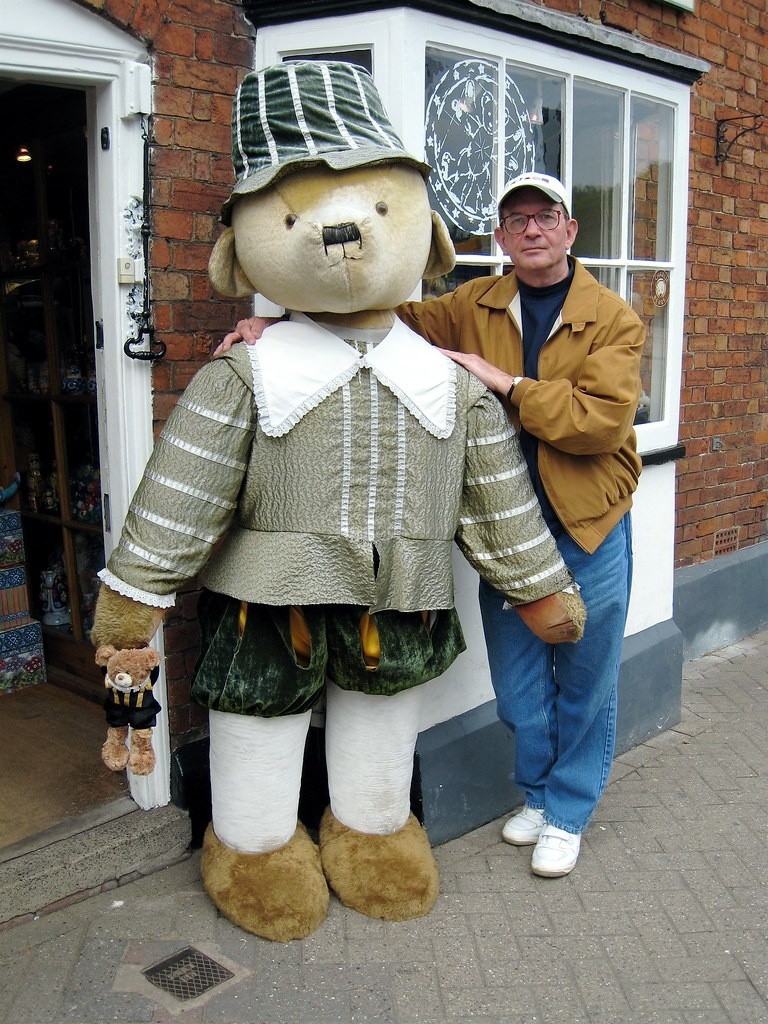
[499, 209, 568, 235]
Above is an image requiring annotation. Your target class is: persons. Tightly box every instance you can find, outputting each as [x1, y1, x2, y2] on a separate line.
[210, 170, 645, 877]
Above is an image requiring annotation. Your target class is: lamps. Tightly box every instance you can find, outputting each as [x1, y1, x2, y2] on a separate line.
[527, 77, 544, 125]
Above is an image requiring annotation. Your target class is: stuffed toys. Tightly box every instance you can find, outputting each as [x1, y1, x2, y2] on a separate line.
[94, 641, 163, 775]
[87, 60, 586, 942]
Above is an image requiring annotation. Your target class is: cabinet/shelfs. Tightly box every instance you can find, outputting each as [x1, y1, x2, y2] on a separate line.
[0, 129, 106, 705]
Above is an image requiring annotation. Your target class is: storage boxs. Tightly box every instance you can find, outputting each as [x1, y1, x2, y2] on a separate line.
[0, 509, 47, 697]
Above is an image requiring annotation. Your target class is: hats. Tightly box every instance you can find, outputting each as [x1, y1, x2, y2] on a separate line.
[497, 172, 571, 221]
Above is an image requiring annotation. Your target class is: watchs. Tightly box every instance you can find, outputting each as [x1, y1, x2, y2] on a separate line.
[506, 376, 525, 399]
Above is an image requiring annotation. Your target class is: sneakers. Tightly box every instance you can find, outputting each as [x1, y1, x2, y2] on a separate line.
[502, 803, 549, 846]
[531, 822, 581, 877]
